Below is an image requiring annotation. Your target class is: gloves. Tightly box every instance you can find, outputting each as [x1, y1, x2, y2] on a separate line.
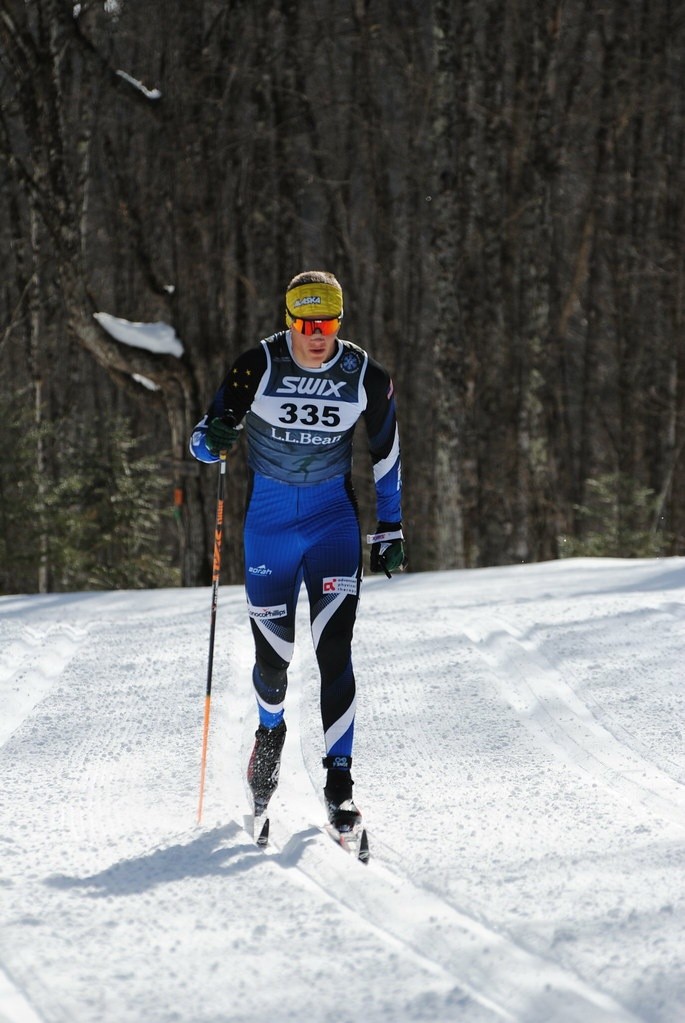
[369, 522, 405, 573]
[207, 413, 241, 460]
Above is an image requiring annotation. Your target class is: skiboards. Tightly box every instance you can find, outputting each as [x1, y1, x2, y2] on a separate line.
[239, 812, 370, 867]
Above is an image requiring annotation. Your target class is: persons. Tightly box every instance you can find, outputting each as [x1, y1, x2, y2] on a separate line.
[189, 271, 404, 833]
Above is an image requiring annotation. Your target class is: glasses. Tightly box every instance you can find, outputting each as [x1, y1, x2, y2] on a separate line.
[292, 317, 339, 336]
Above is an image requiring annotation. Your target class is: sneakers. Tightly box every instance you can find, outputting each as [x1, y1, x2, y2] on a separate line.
[247, 720, 287, 797]
[321, 757, 362, 832]
